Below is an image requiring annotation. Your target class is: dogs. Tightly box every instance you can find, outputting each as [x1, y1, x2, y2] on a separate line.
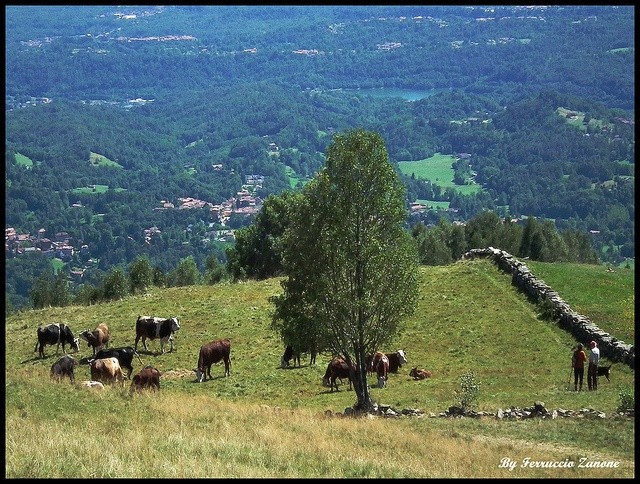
[597, 364, 612, 382]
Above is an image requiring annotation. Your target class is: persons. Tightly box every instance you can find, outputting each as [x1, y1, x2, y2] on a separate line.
[588, 340, 600, 391]
[572, 343, 587, 390]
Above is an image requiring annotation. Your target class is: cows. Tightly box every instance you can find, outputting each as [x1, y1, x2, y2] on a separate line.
[384, 347, 407, 374]
[90, 357, 128, 389]
[330, 362, 357, 391]
[34, 323, 80, 358]
[366, 352, 389, 387]
[408, 366, 431, 381]
[321, 355, 348, 386]
[87, 346, 145, 380]
[129, 365, 161, 397]
[280, 345, 316, 369]
[135, 314, 181, 354]
[78, 323, 110, 353]
[50, 355, 79, 386]
[192, 338, 232, 383]
